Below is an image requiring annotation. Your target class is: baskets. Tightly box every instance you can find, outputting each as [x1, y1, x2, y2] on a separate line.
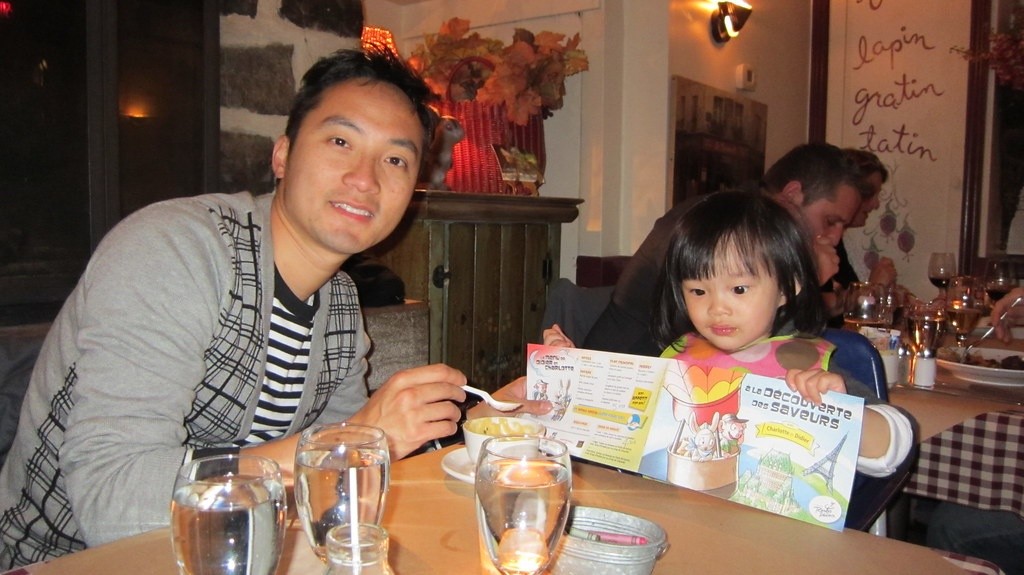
[433, 56, 547, 194]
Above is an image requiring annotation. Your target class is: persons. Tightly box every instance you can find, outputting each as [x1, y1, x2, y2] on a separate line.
[583, 140, 863, 357]
[927, 287, 1024, 568]
[543, 190, 914, 478]
[819, 150, 911, 329]
[0, 42, 552, 575]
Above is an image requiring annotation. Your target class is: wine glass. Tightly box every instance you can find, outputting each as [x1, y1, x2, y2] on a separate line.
[982, 257, 1017, 301]
[927, 252, 954, 297]
[944, 276, 985, 348]
[894, 303, 946, 391]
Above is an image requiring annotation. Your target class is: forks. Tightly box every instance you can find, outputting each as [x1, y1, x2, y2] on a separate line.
[460, 385, 523, 411]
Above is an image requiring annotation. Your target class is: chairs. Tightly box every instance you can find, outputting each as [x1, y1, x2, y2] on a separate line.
[819, 329, 916, 531]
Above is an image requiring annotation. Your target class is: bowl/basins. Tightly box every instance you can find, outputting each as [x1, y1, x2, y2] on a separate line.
[463, 417, 546, 466]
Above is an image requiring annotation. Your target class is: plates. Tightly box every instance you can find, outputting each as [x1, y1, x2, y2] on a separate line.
[440, 447, 571, 486]
[935, 347, 1024, 387]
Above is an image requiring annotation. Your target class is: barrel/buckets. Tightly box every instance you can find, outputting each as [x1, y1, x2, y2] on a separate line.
[548, 506, 669, 575]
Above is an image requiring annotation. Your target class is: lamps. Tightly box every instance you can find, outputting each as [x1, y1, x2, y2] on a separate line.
[711, 2, 752, 43]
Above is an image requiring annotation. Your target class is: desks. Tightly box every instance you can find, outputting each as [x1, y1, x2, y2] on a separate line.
[0, 440, 1008, 575]
[869, 374, 1024, 536]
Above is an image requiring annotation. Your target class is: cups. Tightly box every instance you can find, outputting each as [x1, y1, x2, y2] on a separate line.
[907, 356, 938, 389]
[880, 354, 899, 389]
[293, 422, 391, 564]
[169, 453, 287, 575]
[474, 435, 573, 575]
[843, 282, 885, 332]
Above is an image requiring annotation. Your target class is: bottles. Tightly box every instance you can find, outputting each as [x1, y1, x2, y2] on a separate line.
[320, 522, 396, 575]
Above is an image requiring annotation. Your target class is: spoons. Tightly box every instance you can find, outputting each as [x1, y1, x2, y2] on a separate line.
[963, 297, 1021, 360]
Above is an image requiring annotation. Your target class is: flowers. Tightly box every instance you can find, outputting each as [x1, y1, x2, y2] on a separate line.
[400, 14, 588, 127]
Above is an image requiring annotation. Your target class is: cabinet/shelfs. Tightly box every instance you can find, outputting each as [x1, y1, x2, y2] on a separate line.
[342, 189, 584, 407]
